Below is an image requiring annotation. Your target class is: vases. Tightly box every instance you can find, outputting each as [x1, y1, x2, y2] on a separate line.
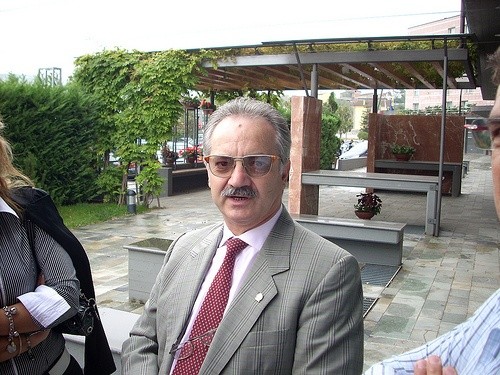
[204, 109, 213, 114]
[186, 155, 196, 163]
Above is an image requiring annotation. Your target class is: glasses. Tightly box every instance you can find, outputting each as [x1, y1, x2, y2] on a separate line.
[465, 117, 500, 151]
[204, 154, 280, 179]
[168, 328, 216, 360]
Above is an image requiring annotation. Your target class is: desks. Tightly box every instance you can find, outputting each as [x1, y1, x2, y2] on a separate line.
[301, 170, 445, 236]
[374, 159, 462, 197]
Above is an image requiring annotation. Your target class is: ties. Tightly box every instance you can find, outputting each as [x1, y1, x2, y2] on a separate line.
[169, 237, 249, 375]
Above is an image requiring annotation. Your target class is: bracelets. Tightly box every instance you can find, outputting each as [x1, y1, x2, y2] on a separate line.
[18, 335, 22, 357]
[2, 305, 19, 354]
[24, 334, 34, 360]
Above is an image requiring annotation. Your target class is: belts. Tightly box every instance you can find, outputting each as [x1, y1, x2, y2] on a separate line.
[46, 343, 70, 375]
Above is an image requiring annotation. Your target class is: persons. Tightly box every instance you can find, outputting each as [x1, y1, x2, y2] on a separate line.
[120, 96, 364, 375]
[362, 43, 500, 375]
[0, 119, 117, 375]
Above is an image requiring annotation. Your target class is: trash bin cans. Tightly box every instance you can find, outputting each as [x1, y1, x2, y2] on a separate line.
[126, 174, 140, 203]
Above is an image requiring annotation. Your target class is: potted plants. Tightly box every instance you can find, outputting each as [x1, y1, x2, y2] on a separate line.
[354, 193, 382, 219]
[162, 148, 179, 165]
[389, 144, 417, 161]
[183, 97, 200, 108]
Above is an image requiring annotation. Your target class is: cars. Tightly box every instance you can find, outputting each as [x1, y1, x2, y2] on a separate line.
[337, 140, 369, 161]
[108, 134, 204, 182]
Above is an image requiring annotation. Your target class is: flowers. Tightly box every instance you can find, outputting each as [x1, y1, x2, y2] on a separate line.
[201, 100, 217, 111]
[179, 144, 202, 159]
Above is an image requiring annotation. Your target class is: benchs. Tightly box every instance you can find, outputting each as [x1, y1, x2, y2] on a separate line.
[125, 238, 172, 302]
[286, 213, 406, 265]
[153, 163, 208, 197]
[63, 306, 141, 375]
